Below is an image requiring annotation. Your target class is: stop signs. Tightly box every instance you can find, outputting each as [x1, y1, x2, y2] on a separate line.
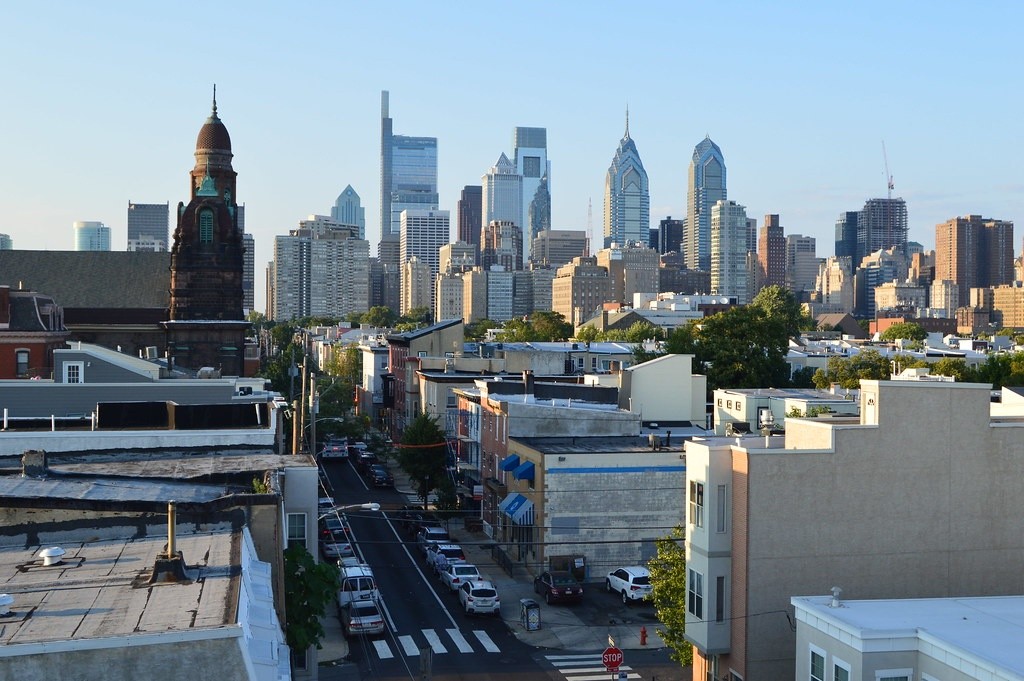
[602, 648, 623, 670]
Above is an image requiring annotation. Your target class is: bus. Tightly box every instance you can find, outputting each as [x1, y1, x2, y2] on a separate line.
[322, 434, 349, 458]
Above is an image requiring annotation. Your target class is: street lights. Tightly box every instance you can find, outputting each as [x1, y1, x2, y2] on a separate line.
[300, 417, 346, 452]
[423, 470, 430, 511]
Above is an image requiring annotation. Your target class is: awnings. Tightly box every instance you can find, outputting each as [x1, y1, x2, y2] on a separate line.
[500, 454, 520, 470]
[510, 461, 535, 479]
[497, 490, 534, 524]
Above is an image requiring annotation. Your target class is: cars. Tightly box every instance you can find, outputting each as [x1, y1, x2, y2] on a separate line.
[318, 496, 360, 567]
[534, 570, 584, 604]
[458, 580, 500, 618]
[340, 600, 385, 639]
[440, 564, 484, 591]
[396, 505, 441, 542]
[353, 440, 395, 489]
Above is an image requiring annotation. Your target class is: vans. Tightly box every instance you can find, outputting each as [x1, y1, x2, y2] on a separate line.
[337, 565, 378, 607]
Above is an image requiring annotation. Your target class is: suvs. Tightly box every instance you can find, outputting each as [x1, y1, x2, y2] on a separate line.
[424, 544, 466, 575]
[417, 527, 451, 554]
[605, 565, 653, 604]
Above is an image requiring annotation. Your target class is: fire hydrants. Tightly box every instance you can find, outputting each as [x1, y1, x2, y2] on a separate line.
[640, 624, 648, 645]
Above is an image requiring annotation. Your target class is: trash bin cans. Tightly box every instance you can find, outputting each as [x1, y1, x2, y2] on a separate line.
[519, 598, 542, 631]
